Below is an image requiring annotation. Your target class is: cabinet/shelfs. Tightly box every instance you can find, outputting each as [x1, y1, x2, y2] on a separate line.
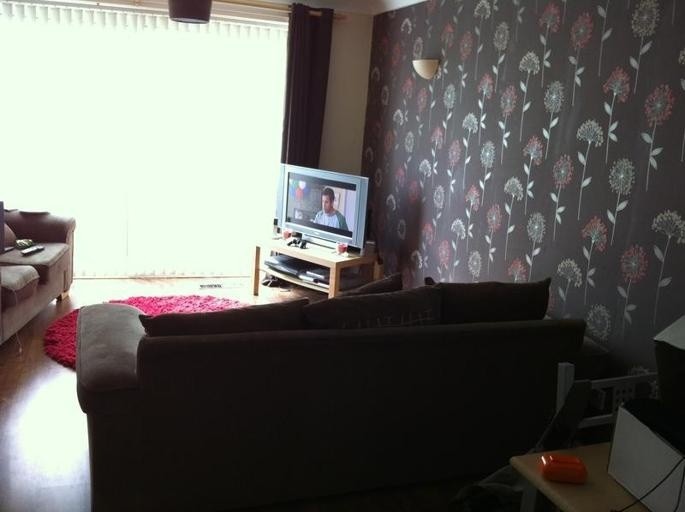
[250, 232, 379, 302]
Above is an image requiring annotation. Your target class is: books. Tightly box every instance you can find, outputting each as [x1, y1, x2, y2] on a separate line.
[306, 268, 329, 279]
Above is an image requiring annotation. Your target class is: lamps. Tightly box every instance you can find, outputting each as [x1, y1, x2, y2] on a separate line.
[411, 57, 440, 81]
[165, 0, 215, 27]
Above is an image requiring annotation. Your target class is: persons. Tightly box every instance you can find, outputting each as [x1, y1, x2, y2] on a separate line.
[314, 187, 348, 231]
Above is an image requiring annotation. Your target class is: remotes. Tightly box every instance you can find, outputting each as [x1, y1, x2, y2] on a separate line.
[21, 245, 44, 254]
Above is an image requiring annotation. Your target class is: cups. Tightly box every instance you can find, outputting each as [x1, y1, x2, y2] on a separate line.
[336, 241, 347, 255]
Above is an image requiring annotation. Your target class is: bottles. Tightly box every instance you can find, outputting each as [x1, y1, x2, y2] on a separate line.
[271, 217, 282, 240]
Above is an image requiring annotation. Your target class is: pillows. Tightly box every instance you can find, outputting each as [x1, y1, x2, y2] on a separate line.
[422, 271, 552, 324]
[302, 281, 436, 329]
[134, 291, 311, 336]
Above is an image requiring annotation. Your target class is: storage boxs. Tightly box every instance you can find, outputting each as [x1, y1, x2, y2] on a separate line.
[608, 308, 685, 511]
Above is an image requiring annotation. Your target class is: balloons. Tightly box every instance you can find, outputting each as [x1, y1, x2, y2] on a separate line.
[289, 179, 310, 201]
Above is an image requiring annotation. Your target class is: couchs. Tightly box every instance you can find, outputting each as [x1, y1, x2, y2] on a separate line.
[1, 200, 77, 350]
[64, 294, 602, 511]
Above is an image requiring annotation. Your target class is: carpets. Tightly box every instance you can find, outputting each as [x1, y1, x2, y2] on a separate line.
[41, 291, 258, 374]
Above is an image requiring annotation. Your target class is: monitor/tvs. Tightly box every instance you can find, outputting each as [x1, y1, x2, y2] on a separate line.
[274, 163, 369, 249]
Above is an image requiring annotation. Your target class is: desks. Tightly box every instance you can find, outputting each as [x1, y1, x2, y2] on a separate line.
[511, 442, 656, 512]
